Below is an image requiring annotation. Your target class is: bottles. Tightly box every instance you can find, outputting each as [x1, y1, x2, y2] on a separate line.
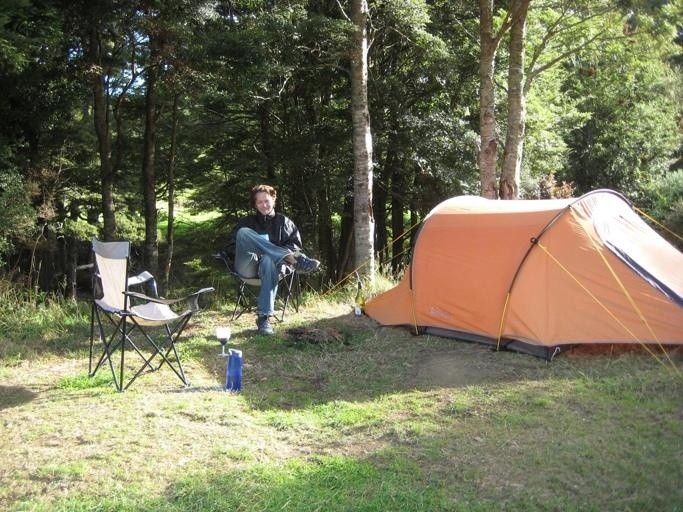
[354, 281, 365, 312]
[225, 346, 243, 393]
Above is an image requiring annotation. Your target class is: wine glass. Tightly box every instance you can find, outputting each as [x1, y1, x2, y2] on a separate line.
[215, 326, 232, 358]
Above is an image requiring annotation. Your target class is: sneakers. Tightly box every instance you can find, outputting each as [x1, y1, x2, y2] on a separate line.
[293, 254, 320, 274]
[256, 318, 272, 335]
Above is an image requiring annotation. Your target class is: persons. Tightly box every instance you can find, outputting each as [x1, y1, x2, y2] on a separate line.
[231, 183, 321, 335]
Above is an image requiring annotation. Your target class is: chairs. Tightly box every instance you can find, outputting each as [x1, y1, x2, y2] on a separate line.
[212, 241, 300, 324]
[87, 233, 215, 394]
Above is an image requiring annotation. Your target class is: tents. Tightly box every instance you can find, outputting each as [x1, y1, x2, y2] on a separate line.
[362, 188, 683, 362]
